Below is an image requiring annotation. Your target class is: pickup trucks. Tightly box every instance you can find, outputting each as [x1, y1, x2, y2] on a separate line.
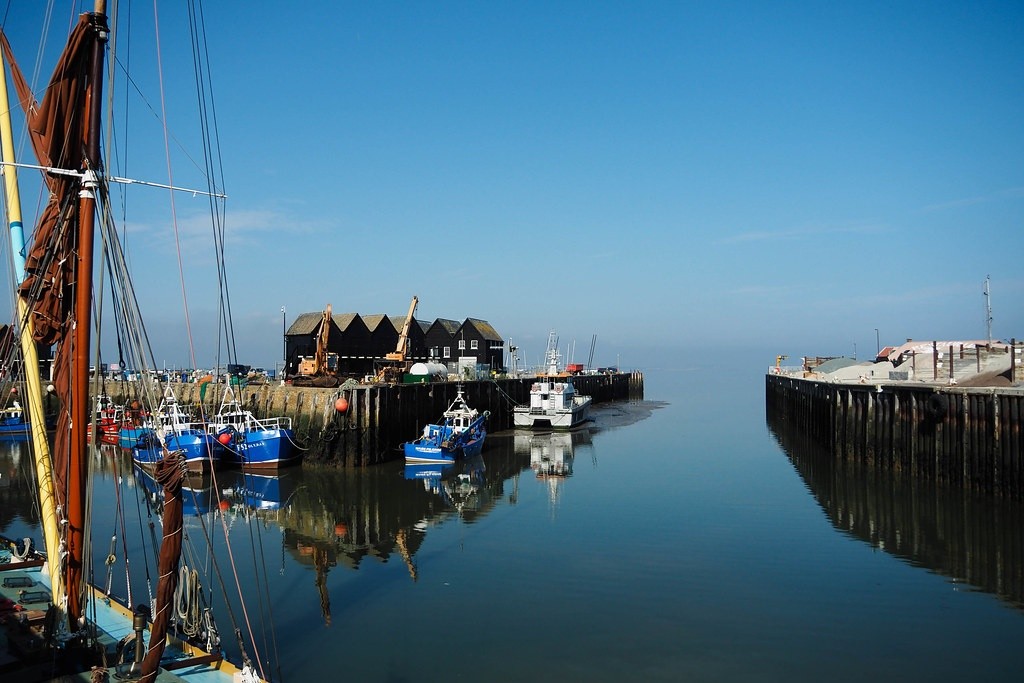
[492, 370, 513, 379]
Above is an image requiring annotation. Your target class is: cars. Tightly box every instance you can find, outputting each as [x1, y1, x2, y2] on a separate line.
[230, 365, 247, 376]
[291, 375, 315, 387]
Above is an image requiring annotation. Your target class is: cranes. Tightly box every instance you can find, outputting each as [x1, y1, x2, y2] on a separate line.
[386, 296, 419, 362]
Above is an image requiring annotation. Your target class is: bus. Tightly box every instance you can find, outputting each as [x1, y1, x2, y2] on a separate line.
[298, 304, 338, 386]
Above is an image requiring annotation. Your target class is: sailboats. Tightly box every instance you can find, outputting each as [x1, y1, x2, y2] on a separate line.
[0, 0, 281, 683]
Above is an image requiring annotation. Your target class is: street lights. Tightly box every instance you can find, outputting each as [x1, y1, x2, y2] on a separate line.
[460, 330, 463, 357]
[875, 329, 879, 354]
[491, 356, 495, 370]
[281, 306, 285, 361]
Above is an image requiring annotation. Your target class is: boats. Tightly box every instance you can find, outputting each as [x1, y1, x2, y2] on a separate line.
[404, 385, 487, 466]
[131, 460, 229, 518]
[218, 467, 294, 524]
[513, 382, 592, 431]
[204, 373, 305, 468]
[0, 399, 31, 432]
[513, 428, 593, 514]
[86, 394, 149, 445]
[131, 375, 232, 474]
[404, 454, 486, 513]
